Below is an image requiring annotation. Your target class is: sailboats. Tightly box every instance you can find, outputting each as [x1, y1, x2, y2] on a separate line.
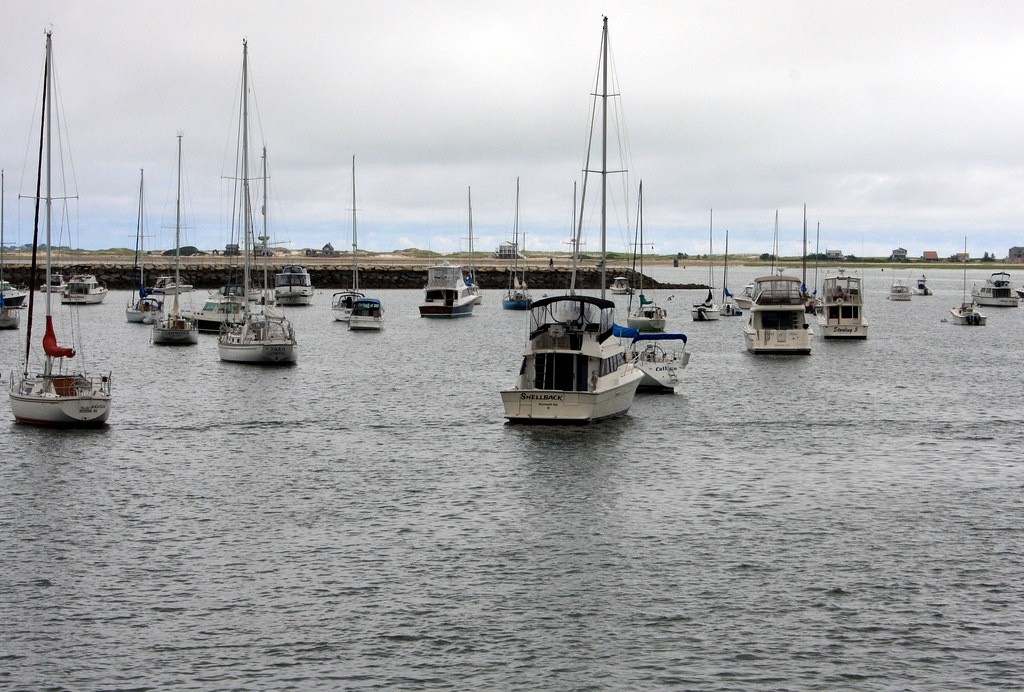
[501, 175, 535, 311]
[2, 26, 364, 430]
[464, 185, 483, 306]
[499, 16, 645, 423]
[950, 235, 988, 327]
[628, 179, 814, 356]
[796, 203, 823, 316]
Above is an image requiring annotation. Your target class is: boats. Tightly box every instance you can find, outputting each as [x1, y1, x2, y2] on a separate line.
[610, 275, 634, 295]
[890, 279, 911, 301]
[970, 272, 1019, 308]
[909, 275, 934, 296]
[61, 274, 109, 305]
[1015, 285, 1024, 301]
[815, 265, 869, 340]
[734, 282, 755, 310]
[40, 272, 68, 293]
[347, 297, 386, 331]
[152, 275, 194, 296]
[418, 265, 478, 320]
[626, 332, 690, 394]
